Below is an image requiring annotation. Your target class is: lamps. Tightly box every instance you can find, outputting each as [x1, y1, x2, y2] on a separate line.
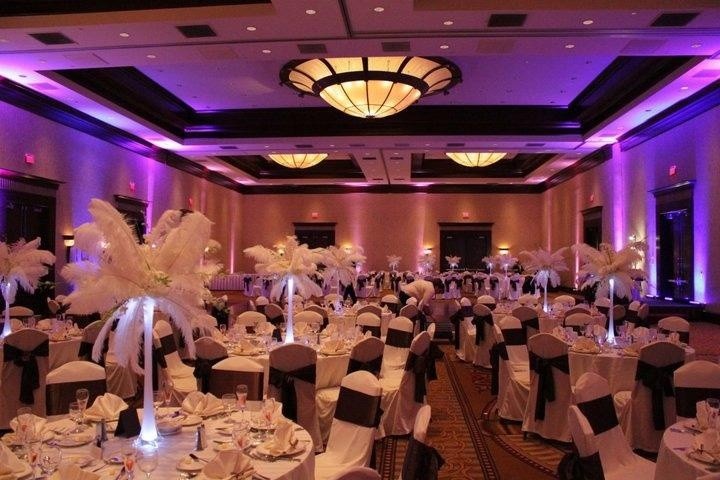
[268, 153, 331, 170]
[277, 57, 461, 122]
[446, 152, 507, 169]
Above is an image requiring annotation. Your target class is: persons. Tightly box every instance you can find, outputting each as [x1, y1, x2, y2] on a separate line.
[398, 278, 444, 329]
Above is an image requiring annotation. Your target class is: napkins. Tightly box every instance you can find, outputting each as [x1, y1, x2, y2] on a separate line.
[83, 392, 129, 421]
[196, 450, 255, 479]
[693, 428, 718, 454]
[9, 413, 48, 439]
[194, 394, 239, 417]
[2, 444, 26, 474]
[263, 418, 297, 456]
[254, 398, 283, 424]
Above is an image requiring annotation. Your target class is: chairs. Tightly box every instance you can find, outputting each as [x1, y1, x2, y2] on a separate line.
[313, 337, 384, 446]
[311, 368, 383, 479]
[211, 356, 264, 402]
[331, 466, 383, 480]
[673, 359, 720, 423]
[614, 340, 687, 452]
[557, 406, 606, 478]
[2, 270, 690, 373]
[151, 328, 198, 408]
[574, 371, 656, 479]
[520, 334, 576, 442]
[375, 331, 432, 440]
[491, 325, 530, 421]
[44, 361, 108, 417]
[192, 337, 228, 400]
[267, 343, 325, 452]
[1, 327, 51, 430]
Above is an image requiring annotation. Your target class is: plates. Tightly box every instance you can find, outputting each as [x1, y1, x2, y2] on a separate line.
[1, 384, 303, 479]
[667, 397, 720, 471]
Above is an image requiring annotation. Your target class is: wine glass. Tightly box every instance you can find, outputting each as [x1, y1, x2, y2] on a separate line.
[22, 313, 84, 342]
[492, 298, 686, 362]
[217, 313, 374, 358]
[287, 293, 353, 314]
